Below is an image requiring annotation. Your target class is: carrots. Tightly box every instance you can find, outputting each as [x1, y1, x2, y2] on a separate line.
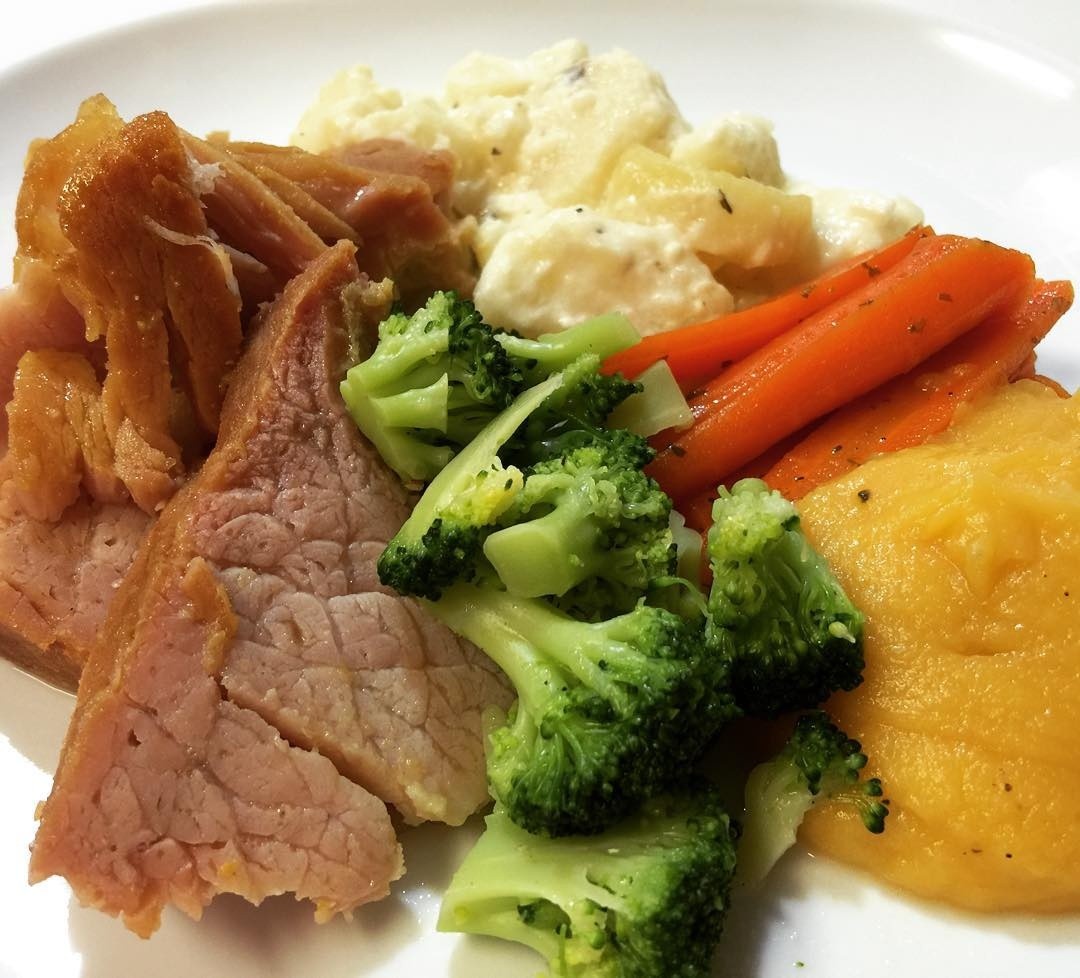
[601, 223, 1076, 502]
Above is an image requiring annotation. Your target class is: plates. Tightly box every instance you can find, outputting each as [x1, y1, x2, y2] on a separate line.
[2, 0, 1079, 978]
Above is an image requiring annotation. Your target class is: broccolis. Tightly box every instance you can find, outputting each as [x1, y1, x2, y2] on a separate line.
[338, 294, 891, 978]
